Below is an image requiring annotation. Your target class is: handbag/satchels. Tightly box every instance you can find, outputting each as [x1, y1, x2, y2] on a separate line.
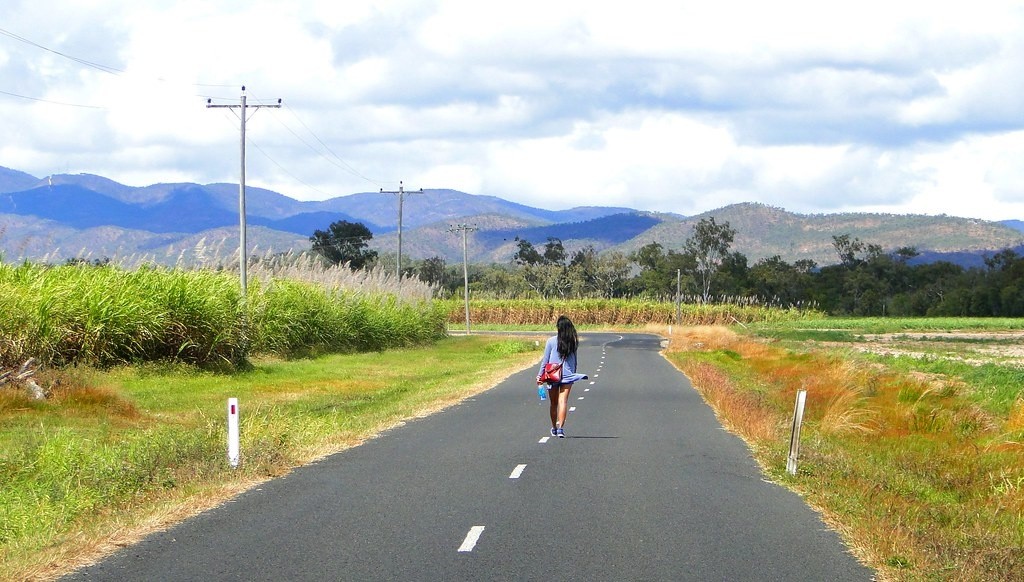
[540, 362, 563, 383]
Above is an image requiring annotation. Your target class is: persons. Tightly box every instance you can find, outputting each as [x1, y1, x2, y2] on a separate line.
[537, 316, 579, 438]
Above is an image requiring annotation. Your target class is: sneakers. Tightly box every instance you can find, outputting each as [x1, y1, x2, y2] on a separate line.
[557, 428, 565, 437]
[550, 427, 557, 436]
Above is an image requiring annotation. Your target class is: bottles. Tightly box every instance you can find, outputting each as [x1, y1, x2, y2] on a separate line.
[538, 382, 547, 401]
